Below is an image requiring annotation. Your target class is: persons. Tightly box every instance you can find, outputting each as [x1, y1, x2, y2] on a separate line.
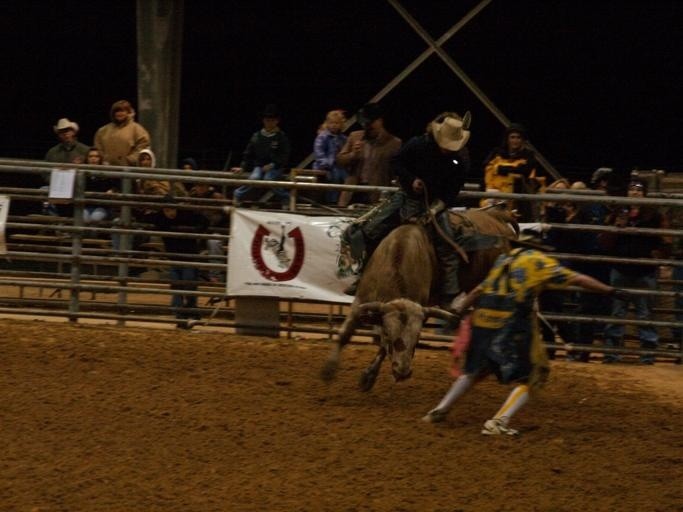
[346, 117, 471, 297]
[312, 109, 404, 210]
[44, 100, 225, 310]
[477, 128, 666, 364]
[233, 109, 284, 201]
[420, 227, 636, 440]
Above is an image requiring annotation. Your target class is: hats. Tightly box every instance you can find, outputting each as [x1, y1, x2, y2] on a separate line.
[54, 119, 78, 131]
[519, 223, 560, 251]
[431, 118, 470, 151]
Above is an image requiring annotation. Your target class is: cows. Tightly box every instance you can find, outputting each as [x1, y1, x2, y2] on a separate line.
[320, 202, 520, 392]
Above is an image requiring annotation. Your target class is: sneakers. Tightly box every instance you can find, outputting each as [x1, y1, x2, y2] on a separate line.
[481, 418, 520, 437]
[422, 409, 447, 422]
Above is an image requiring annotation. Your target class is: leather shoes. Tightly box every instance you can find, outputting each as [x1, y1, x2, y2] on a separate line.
[343, 280, 359, 294]
